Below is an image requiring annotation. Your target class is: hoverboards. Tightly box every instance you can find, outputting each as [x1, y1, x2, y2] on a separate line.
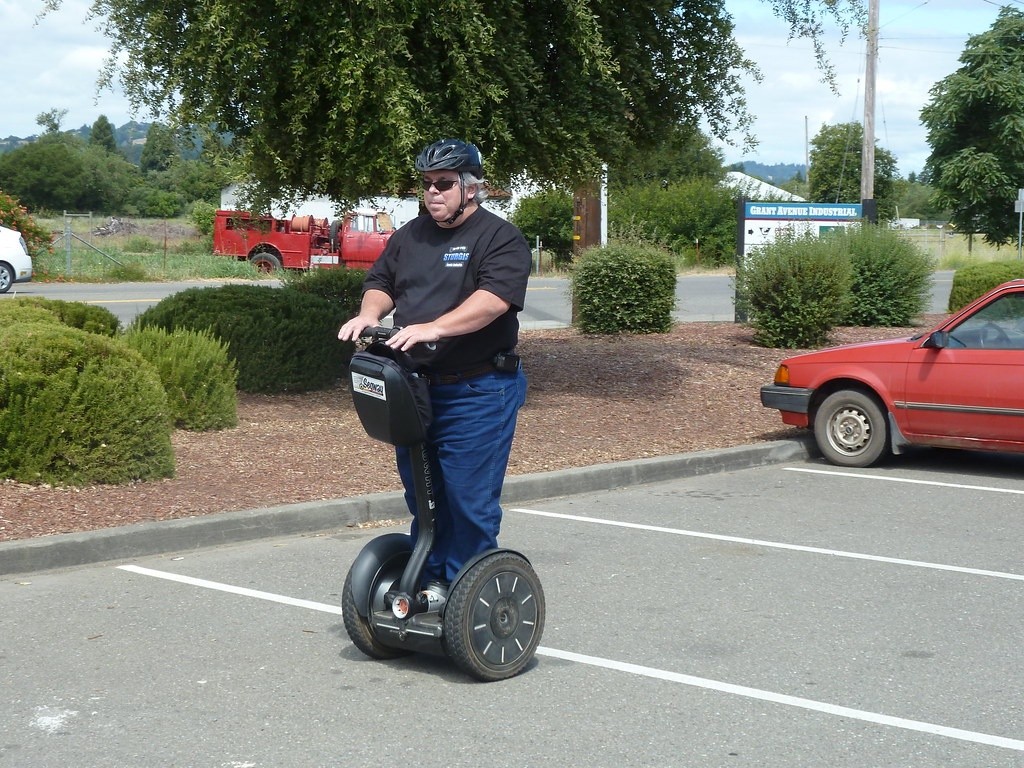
[341, 321, 549, 676]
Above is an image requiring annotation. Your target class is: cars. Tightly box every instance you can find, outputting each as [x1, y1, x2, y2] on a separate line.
[0, 223, 32, 295]
[757, 278, 1023, 472]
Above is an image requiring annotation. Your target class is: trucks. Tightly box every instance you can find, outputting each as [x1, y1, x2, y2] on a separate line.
[205, 206, 405, 276]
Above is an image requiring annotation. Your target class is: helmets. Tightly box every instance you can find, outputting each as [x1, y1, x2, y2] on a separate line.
[414, 138, 482, 179]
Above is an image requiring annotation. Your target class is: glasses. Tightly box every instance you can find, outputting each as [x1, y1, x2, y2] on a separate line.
[423, 181, 459, 191]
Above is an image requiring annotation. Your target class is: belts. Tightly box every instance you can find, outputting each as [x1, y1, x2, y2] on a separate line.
[425, 362, 496, 387]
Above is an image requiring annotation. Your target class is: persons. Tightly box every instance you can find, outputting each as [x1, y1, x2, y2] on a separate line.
[337, 138, 532, 616]
